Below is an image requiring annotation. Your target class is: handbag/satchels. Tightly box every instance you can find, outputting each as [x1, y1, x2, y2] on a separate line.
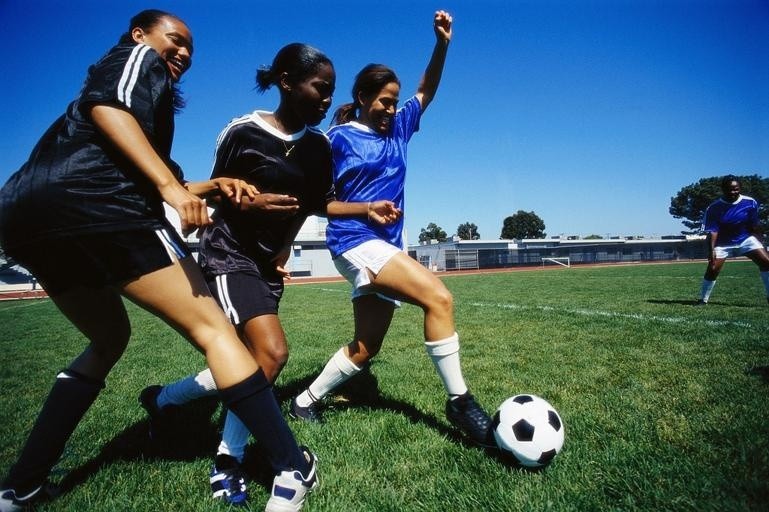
[367, 197, 373, 227]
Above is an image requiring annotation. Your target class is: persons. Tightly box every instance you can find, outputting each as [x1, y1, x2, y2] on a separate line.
[136, 45, 402, 505]
[1, 9, 321, 511]
[700, 174, 768, 306]
[291, 10, 503, 454]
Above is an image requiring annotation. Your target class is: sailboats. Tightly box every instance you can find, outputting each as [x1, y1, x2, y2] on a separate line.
[491, 394, 565, 468]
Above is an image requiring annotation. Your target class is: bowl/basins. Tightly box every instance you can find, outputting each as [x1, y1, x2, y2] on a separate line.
[273, 108, 302, 157]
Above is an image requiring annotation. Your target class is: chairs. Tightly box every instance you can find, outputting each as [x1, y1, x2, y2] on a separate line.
[210, 457, 248, 503]
[139, 385, 162, 416]
[289, 400, 324, 423]
[446, 393, 499, 448]
[0, 486, 42, 512]
[265, 446, 320, 512]
